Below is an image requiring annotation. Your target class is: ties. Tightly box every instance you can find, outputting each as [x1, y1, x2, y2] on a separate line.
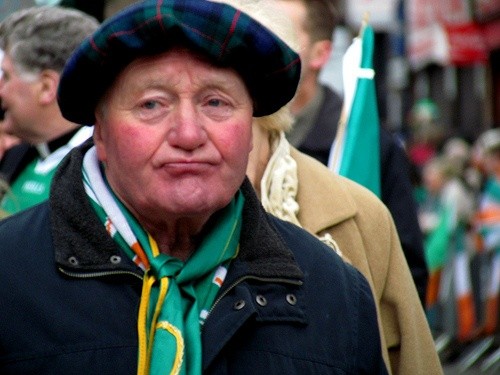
[82, 145, 246, 375]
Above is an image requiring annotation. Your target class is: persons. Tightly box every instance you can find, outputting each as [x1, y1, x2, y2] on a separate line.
[0, 0, 500, 375]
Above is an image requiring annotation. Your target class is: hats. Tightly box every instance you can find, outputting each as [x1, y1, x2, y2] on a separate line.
[56, 0, 302, 127]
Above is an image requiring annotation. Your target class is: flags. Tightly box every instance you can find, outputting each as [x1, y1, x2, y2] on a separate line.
[326, 12, 382, 200]
